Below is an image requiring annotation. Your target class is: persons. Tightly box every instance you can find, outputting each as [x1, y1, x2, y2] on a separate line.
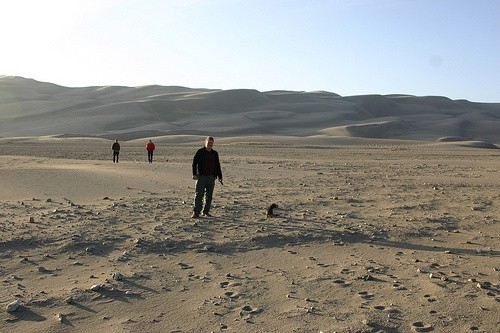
[145, 139, 155, 163]
[191, 136, 223, 218]
[111, 139, 121, 163]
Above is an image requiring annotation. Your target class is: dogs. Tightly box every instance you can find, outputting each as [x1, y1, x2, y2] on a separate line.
[266, 203, 280, 218]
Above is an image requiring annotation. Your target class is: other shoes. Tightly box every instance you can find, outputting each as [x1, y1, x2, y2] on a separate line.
[203, 213, 211, 217]
[192, 213, 199, 218]
